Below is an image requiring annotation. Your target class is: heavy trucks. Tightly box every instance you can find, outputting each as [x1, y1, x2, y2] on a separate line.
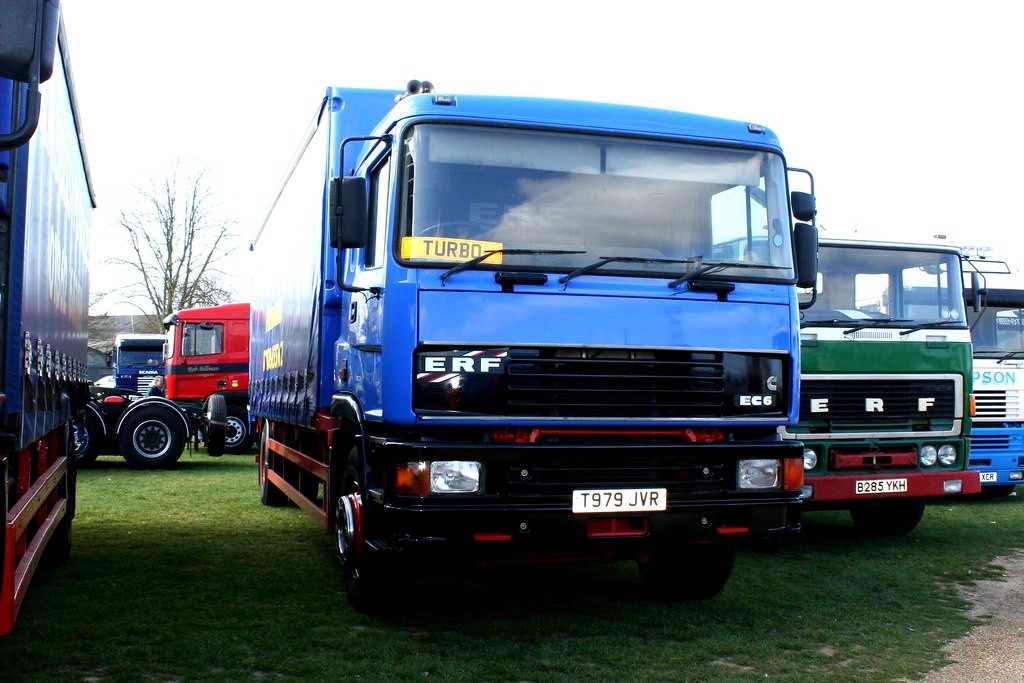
[161, 301, 255, 453]
[105, 334, 166, 398]
[72, 386, 237, 471]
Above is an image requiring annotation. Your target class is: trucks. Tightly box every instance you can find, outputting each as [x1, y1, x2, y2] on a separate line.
[246, 84, 818, 618]
[714, 230, 988, 544]
[1, 0, 99, 637]
[855, 265, 1024, 503]
